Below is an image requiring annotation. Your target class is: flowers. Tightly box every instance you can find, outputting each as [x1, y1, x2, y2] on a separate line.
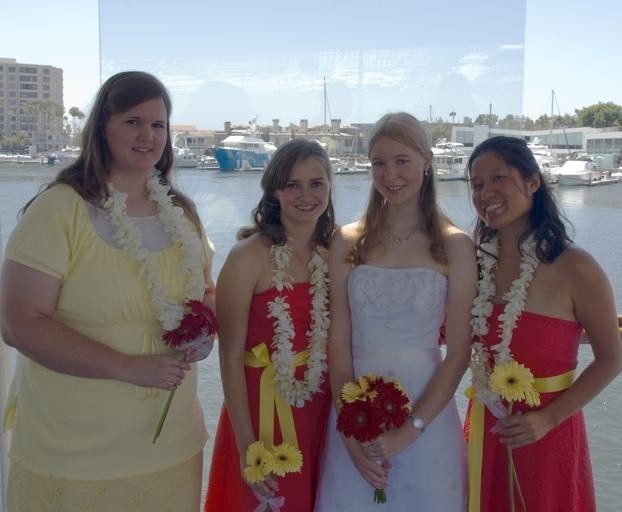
[152, 299, 217, 442]
[244, 441, 303, 512]
[489, 360, 542, 512]
[266, 237, 330, 406]
[337, 375, 412, 501]
[105, 172, 206, 330]
[470, 228, 540, 404]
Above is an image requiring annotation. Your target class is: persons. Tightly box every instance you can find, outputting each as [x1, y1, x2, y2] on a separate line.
[460, 136, 620, 511]
[308, 112, 479, 512]
[204, 139, 342, 512]
[0, 70, 220, 511]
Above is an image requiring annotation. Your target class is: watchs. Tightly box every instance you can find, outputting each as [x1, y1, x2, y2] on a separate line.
[407, 412, 425, 433]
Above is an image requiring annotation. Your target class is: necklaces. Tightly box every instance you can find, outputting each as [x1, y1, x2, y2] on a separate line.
[383, 221, 423, 243]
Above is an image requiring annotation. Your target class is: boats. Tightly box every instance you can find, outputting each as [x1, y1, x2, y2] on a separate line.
[54, 142, 82, 164]
